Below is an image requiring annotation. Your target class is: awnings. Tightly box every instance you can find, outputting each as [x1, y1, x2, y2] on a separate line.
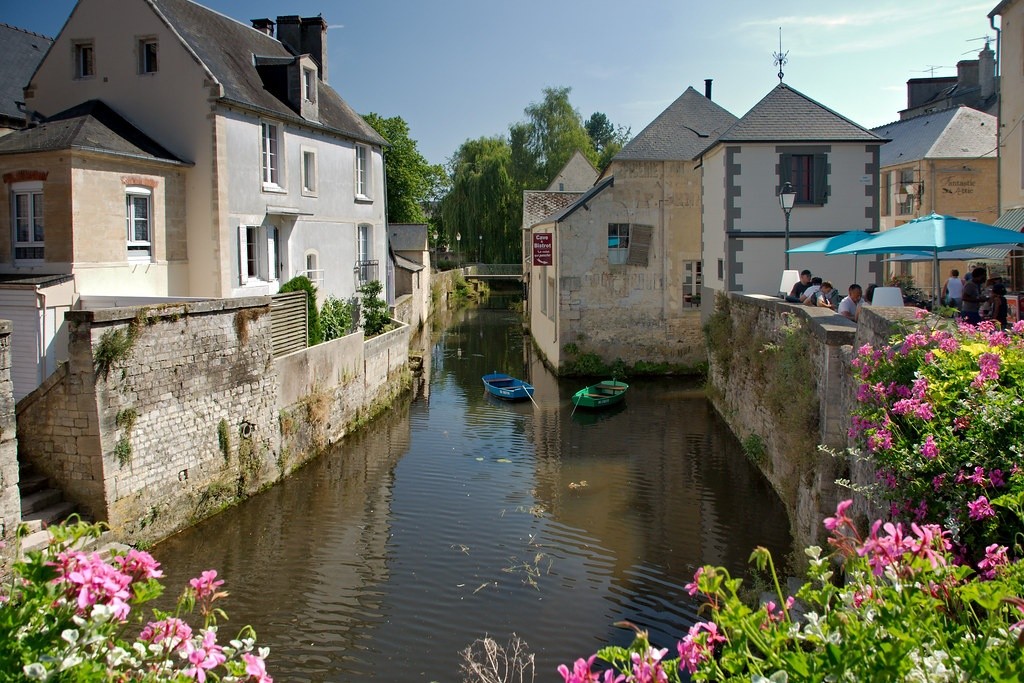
[967, 209, 1024, 259]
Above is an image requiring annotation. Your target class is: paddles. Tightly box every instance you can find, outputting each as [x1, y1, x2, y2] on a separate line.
[570, 394, 582, 417]
[522, 385, 541, 410]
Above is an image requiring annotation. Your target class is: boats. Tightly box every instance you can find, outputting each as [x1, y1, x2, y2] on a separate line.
[572, 378, 629, 407]
[482, 371, 535, 401]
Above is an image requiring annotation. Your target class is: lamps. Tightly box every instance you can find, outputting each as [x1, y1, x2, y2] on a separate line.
[779, 270, 800, 296]
[894, 181, 924, 203]
[871, 286, 904, 306]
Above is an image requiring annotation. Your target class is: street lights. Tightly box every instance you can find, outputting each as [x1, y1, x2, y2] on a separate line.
[779, 182, 797, 270]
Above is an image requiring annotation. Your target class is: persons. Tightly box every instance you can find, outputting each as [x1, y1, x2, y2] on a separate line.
[941, 264, 1008, 332]
[789, 270, 878, 324]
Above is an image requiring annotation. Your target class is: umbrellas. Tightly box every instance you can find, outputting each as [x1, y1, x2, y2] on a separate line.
[825, 210, 1024, 307]
[880, 250, 994, 311]
[784, 230, 877, 285]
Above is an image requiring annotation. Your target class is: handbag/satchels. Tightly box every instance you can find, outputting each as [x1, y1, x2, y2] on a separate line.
[811, 293, 817, 306]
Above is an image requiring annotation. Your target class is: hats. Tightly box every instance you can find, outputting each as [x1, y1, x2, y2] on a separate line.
[801, 270, 811, 275]
